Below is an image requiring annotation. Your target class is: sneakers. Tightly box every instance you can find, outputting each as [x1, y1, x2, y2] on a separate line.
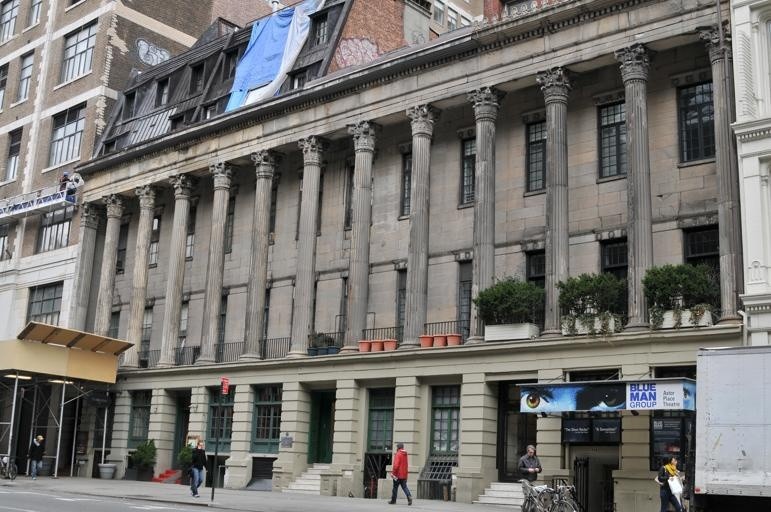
[408, 496, 412, 505]
[388, 501, 396, 504]
[33, 476, 36, 480]
[192, 492, 200, 497]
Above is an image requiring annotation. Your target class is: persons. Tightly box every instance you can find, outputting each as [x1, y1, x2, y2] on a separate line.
[59, 172, 71, 191]
[186, 441, 209, 498]
[26, 435, 48, 479]
[518, 445, 543, 509]
[658, 454, 686, 512]
[518, 385, 625, 412]
[388, 442, 412, 505]
[682, 378, 696, 411]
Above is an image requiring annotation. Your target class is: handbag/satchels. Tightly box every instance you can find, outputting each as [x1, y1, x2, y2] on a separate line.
[655, 475, 666, 486]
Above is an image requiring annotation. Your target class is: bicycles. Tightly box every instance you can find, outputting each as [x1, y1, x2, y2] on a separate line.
[0, 454, 17, 479]
[520, 479, 576, 512]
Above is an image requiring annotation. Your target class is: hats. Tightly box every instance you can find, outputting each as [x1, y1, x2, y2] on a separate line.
[398, 443, 403, 448]
[37, 436, 44, 441]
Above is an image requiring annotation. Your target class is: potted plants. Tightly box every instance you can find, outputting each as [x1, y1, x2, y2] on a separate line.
[175, 443, 197, 485]
[307, 330, 344, 356]
[469, 263, 722, 343]
[127, 438, 159, 481]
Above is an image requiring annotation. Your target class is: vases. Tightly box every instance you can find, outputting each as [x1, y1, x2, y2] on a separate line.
[98, 463, 117, 479]
[418, 333, 463, 349]
[356, 338, 398, 352]
